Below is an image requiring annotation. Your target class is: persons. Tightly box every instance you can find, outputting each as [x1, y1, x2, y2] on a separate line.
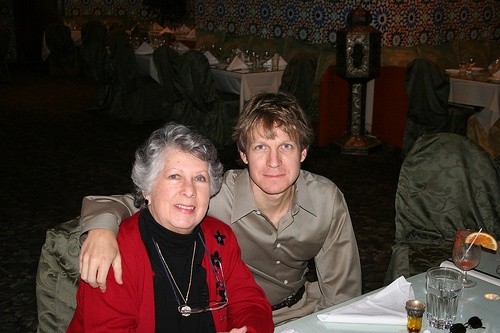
[64, 122, 274, 333]
[79, 90, 362, 327]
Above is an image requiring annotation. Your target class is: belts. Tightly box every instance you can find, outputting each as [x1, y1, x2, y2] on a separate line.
[271, 284, 305, 311]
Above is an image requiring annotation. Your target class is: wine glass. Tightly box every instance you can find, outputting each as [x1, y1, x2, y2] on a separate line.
[452, 227, 481, 288]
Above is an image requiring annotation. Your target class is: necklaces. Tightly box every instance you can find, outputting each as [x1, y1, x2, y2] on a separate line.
[154, 240, 196, 317]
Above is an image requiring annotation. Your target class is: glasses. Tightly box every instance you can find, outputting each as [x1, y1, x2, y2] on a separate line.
[449, 316, 485, 333]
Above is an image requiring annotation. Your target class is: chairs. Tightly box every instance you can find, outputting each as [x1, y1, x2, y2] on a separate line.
[81, 22, 239, 150]
[277, 55, 317, 108]
[382, 132, 500, 285]
[405, 59, 472, 148]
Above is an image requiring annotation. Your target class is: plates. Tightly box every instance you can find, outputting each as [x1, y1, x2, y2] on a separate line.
[468, 67, 485, 71]
[446, 68, 459, 75]
[487, 77, 500, 83]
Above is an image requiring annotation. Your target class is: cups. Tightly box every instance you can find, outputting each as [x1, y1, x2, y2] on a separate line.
[425, 267, 463, 331]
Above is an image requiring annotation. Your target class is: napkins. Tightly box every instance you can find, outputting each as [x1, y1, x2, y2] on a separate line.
[185, 29, 196, 38]
[227, 56, 248, 70]
[317, 275, 415, 325]
[160, 27, 172, 34]
[264, 53, 288, 70]
[151, 23, 163, 31]
[172, 42, 190, 51]
[203, 51, 219, 65]
[134, 42, 154, 54]
[179, 24, 190, 33]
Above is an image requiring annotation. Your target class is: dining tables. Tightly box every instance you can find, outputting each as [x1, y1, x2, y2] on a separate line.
[274, 243, 500, 333]
[136, 54, 284, 114]
[450, 71, 500, 112]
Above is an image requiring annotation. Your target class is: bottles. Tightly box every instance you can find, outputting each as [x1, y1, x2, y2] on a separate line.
[404, 299, 426, 333]
[226, 48, 271, 73]
